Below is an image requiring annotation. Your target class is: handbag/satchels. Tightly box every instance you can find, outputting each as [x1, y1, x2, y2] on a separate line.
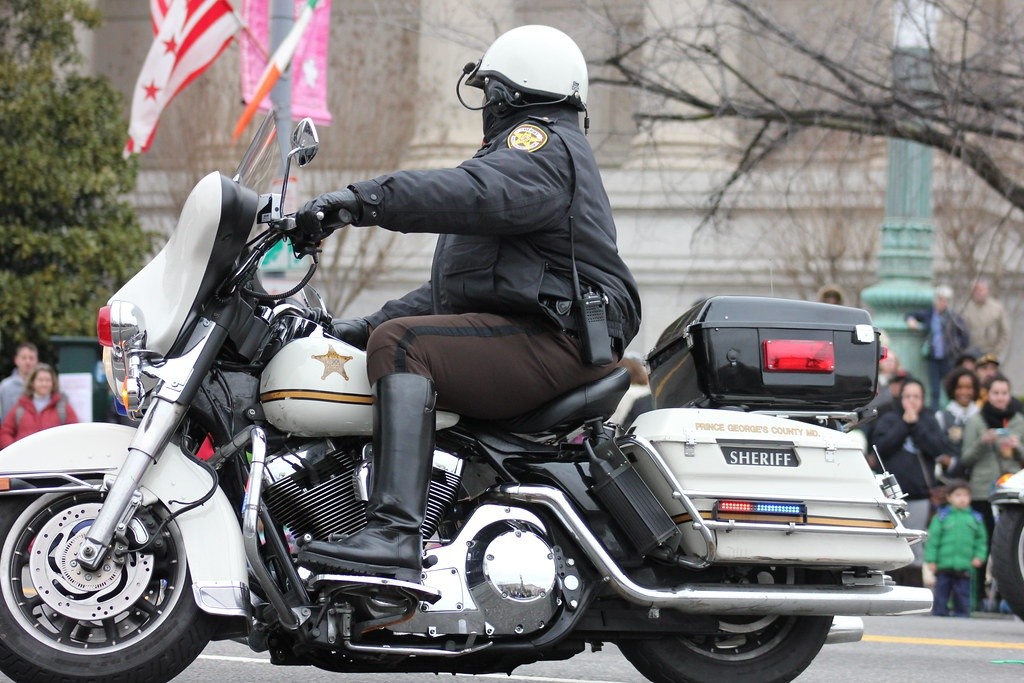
[929, 486, 946, 506]
[921, 338, 931, 357]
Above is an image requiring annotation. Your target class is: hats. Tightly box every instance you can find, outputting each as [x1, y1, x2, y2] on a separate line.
[975, 354, 1000, 369]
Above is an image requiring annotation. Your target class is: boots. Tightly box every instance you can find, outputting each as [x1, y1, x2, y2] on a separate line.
[299, 372, 438, 583]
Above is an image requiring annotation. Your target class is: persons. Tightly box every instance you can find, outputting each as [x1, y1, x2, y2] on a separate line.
[818, 277, 1024, 617]
[0, 342, 78, 449]
[286, 26, 641, 585]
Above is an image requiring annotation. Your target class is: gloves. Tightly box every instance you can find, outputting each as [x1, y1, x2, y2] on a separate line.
[292, 187, 361, 255]
[332, 318, 369, 351]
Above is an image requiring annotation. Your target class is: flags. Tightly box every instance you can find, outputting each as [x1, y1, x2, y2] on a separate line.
[121, 0, 248, 159]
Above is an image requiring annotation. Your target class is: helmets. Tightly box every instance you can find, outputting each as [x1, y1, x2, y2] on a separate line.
[465, 25, 589, 111]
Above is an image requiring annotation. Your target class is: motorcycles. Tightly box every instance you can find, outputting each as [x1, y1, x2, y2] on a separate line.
[0, 108, 934, 682]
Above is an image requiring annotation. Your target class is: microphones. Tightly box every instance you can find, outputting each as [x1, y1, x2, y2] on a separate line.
[456, 62, 503, 111]
